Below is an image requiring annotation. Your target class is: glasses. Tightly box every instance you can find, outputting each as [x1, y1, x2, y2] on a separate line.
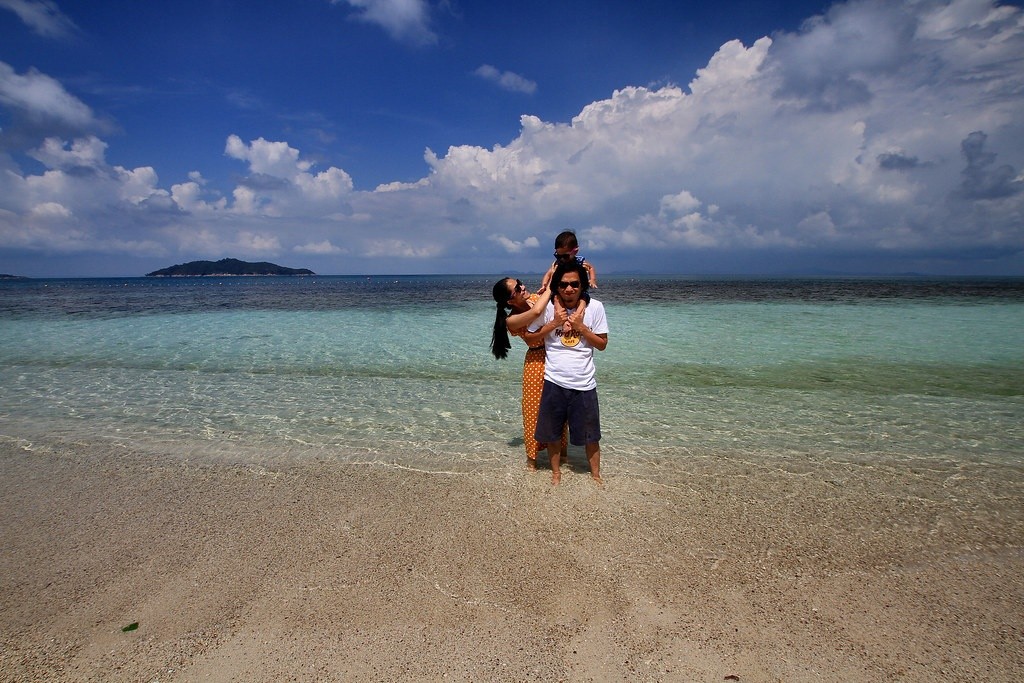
[510, 278, 523, 301]
[553, 247, 577, 259]
[558, 281, 581, 288]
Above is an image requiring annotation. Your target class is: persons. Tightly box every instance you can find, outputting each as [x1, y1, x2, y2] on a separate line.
[490, 260, 568, 468]
[536, 231, 597, 332]
[523, 260, 608, 476]
